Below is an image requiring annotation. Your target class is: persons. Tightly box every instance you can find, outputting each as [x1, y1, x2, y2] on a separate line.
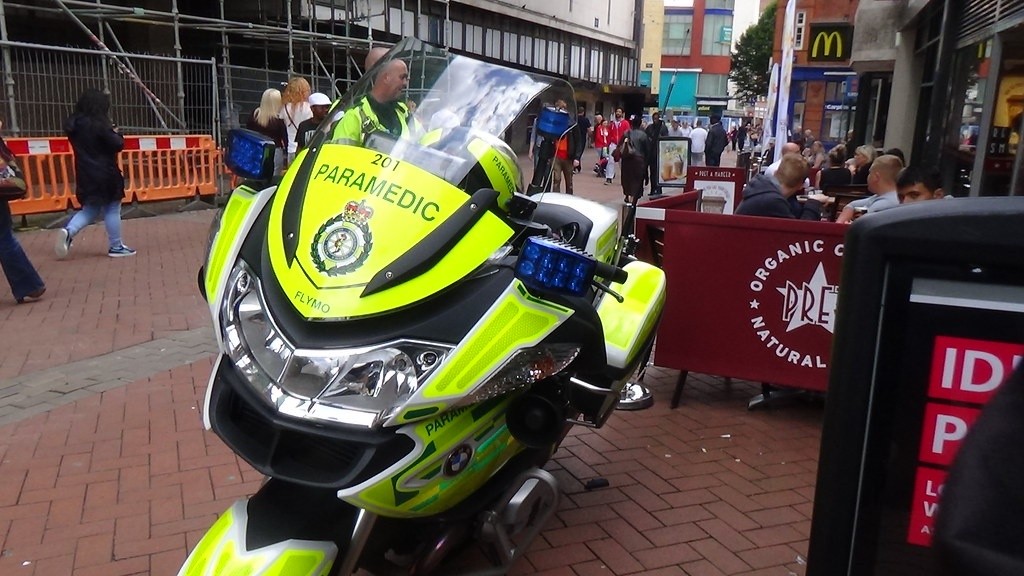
[53, 88, 137, 260]
[247, 46, 462, 174]
[0, 115, 46, 303]
[528, 96, 954, 224]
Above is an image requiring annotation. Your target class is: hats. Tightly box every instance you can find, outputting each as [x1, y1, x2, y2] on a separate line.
[308, 92, 333, 106]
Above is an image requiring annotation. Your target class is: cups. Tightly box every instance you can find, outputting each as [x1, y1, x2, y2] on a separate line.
[815, 189, 822, 195]
[661, 164, 672, 179]
[674, 161, 683, 175]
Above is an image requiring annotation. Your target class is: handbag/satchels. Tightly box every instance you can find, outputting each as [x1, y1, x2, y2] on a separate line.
[1, 177, 28, 201]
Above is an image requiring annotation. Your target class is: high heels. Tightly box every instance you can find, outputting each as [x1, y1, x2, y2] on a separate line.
[17, 287, 47, 304]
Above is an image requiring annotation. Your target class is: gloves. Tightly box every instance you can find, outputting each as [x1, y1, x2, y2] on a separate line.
[612, 149, 622, 162]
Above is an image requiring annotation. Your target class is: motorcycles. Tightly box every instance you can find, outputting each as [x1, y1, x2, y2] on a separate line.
[174, 36, 673, 576]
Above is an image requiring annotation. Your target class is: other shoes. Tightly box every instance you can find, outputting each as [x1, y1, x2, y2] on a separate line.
[107, 245, 137, 257]
[53, 228, 71, 259]
[624, 198, 629, 203]
[645, 177, 650, 186]
[604, 178, 613, 185]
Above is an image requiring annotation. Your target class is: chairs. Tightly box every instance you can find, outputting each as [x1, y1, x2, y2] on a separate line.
[668, 182, 874, 409]
